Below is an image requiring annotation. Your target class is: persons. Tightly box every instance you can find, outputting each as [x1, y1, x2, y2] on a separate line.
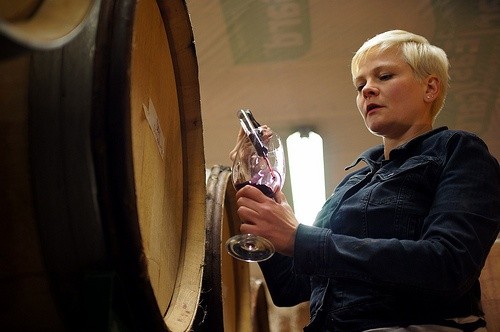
[235, 30, 500, 332]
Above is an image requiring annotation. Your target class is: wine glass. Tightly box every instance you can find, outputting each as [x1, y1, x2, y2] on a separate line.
[225, 128, 287, 264]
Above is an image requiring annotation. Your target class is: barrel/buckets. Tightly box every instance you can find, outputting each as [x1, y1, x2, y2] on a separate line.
[190, 164, 252, 332]
[0, 0, 206, 332]
